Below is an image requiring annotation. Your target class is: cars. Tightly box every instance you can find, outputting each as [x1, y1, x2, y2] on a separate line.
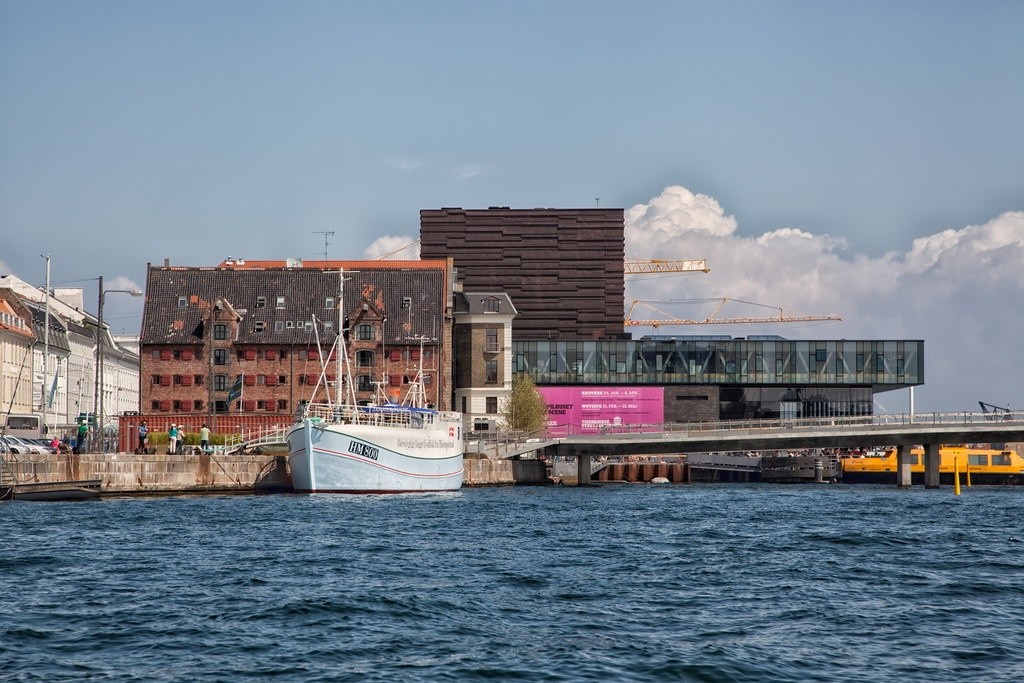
[1, 433, 73, 455]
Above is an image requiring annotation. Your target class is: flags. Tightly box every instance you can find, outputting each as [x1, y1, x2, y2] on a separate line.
[191, 316, 205, 340]
[48, 370, 58, 408]
[335, 318, 350, 341]
[226, 373, 243, 404]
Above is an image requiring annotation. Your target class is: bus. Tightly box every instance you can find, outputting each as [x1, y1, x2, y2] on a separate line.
[5, 414, 47, 441]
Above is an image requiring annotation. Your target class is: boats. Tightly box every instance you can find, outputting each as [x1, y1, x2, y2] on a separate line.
[841, 445, 1024, 486]
[284, 261, 465, 496]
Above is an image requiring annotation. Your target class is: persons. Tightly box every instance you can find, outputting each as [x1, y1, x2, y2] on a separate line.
[718, 441, 926, 467]
[62, 433, 70, 448]
[198, 423, 211, 450]
[137, 419, 149, 454]
[168, 423, 177, 454]
[51, 436, 61, 454]
[74, 421, 92, 453]
[175, 425, 186, 455]
[534, 452, 659, 466]
